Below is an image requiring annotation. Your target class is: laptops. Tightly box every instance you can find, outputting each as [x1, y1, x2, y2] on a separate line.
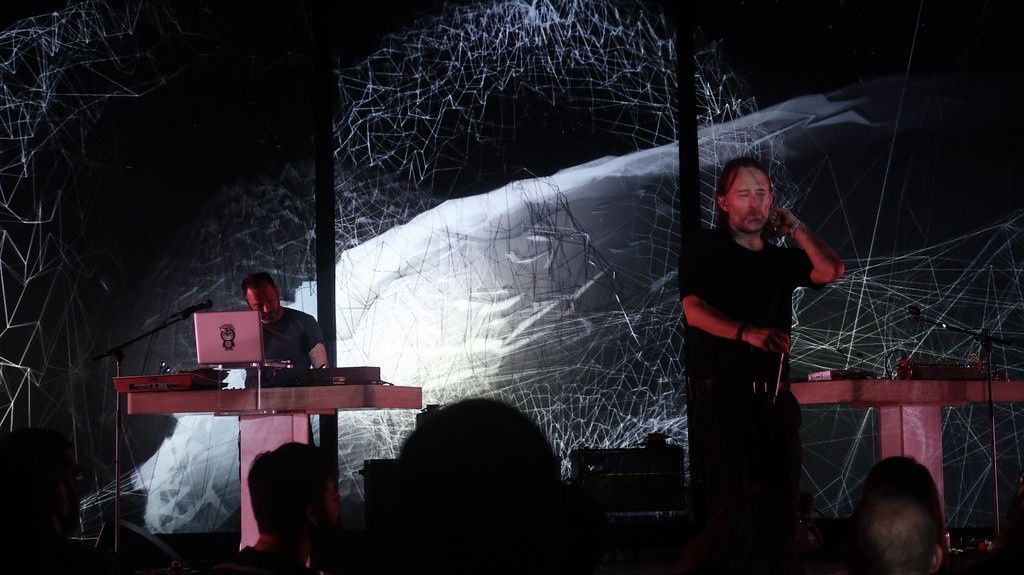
[193, 309, 290, 364]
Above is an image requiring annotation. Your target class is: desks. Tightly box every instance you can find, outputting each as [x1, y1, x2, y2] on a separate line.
[129, 384, 424, 548]
[791, 381, 1024, 520]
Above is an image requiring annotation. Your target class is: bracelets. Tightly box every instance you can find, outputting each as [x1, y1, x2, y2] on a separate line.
[789, 217, 805, 239]
[735, 322, 750, 341]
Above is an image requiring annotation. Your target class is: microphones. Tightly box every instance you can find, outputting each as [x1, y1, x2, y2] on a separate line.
[767, 350, 784, 403]
[169, 299, 214, 319]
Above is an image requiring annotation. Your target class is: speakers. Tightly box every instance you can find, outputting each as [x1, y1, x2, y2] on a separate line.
[571, 444, 687, 517]
[94, 518, 183, 571]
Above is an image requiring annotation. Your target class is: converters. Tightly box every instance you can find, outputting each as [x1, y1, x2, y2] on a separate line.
[647, 432, 668, 445]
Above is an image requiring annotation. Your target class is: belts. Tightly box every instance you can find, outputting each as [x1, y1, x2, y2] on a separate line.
[694, 375, 788, 395]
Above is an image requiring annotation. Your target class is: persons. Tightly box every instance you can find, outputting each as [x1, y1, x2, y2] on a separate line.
[214, 272, 330, 534]
[672, 484, 807, 575]
[217, 441, 340, 575]
[854, 455, 946, 575]
[676, 158, 845, 504]
[393, 398, 562, 575]
[0, 425, 81, 575]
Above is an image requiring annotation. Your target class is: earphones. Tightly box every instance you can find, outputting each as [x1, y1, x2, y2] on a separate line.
[722, 200, 727, 207]
